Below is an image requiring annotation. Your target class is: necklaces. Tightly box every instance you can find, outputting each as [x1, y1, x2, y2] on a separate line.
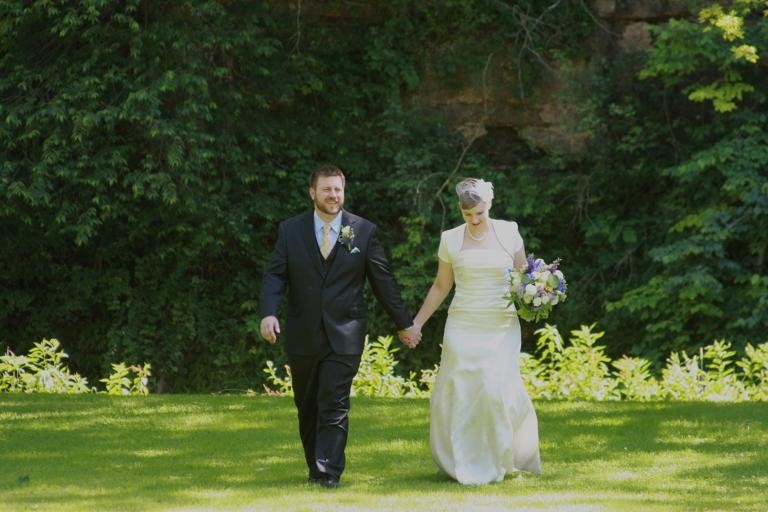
[466, 223, 491, 242]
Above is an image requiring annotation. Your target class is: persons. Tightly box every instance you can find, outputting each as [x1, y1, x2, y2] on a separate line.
[403, 177, 544, 484]
[261, 166, 420, 487]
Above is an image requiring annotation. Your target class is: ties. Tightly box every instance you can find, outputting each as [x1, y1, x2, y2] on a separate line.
[320, 225, 332, 260]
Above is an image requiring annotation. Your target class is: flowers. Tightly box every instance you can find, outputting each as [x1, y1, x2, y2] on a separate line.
[501, 251, 569, 325]
[333, 221, 356, 253]
